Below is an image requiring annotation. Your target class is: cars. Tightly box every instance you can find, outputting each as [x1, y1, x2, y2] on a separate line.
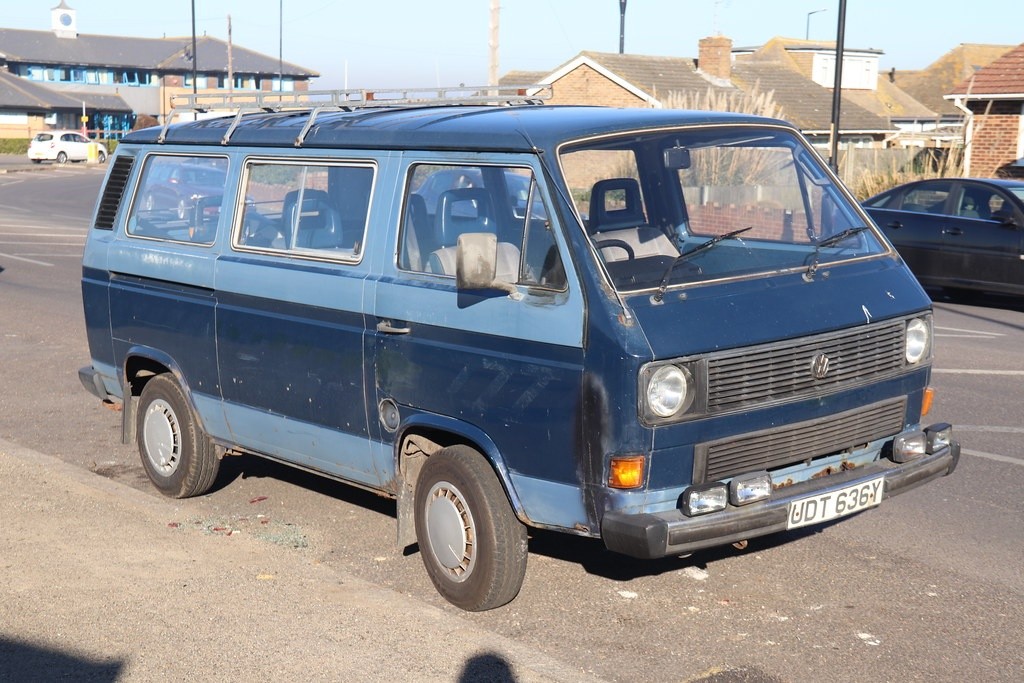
[144, 162, 256, 222]
[829, 176, 1024, 299]
[27, 131, 108, 165]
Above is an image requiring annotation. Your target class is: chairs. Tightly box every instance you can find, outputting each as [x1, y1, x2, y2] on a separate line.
[282, 190, 352, 258]
[430, 186, 537, 286]
[589, 178, 700, 284]
[407, 193, 433, 243]
[907, 196, 998, 220]
[193, 195, 258, 246]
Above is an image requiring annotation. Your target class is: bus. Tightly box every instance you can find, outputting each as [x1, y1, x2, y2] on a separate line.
[76, 87, 962, 615]
